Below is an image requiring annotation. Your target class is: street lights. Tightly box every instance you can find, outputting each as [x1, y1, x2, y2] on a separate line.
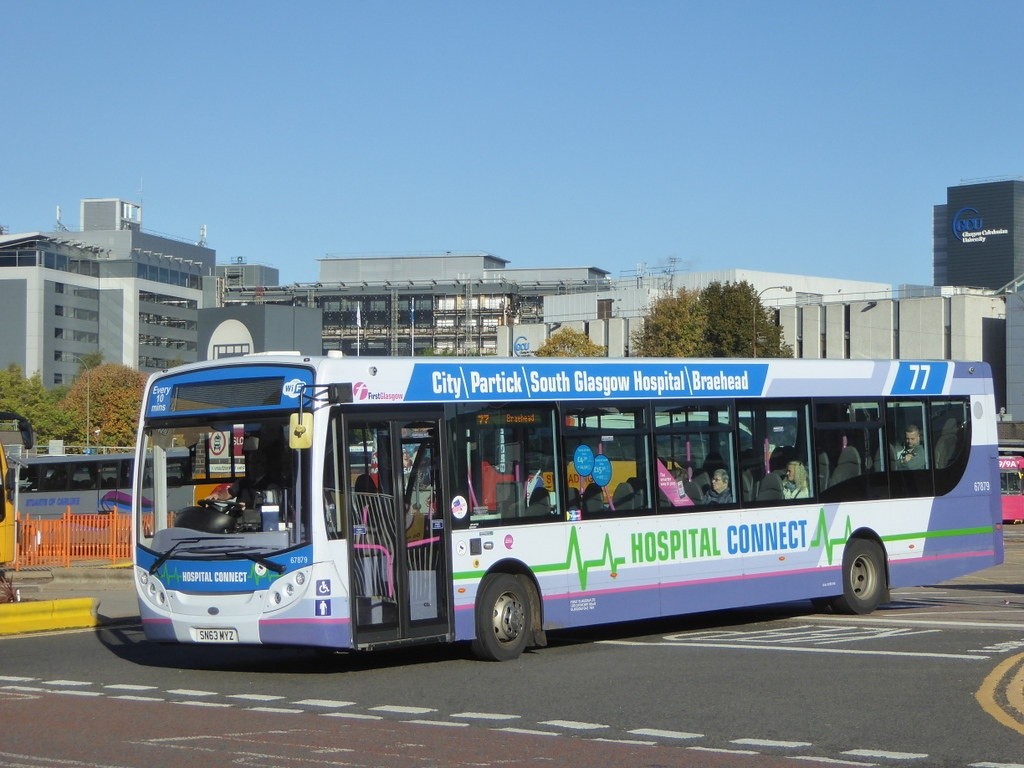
[753, 286, 792, 359]
[62, 352, 90, 447]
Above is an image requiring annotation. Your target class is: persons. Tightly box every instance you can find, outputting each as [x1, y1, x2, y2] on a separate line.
[781, 460, 809, 500]
[704, 468, 733, 504]
[896, 425, 924, 471]
[197, 453, 293, 518]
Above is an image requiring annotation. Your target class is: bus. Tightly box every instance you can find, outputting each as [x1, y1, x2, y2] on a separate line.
[0, 412, 33, 565]
[131, 350, 1004, 662]
[8, 448, 194, 548]
[998, 457, 1023, 520]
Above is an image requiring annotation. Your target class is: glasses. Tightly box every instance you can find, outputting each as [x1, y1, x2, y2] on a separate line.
[712, 478, 721, 482]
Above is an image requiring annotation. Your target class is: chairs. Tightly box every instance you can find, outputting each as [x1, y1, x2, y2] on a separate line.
[527, 414, 959, 515]
[52, 475, 180, 488]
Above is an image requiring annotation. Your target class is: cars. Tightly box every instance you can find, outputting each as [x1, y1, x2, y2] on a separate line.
[349, 445, 372, 468]
